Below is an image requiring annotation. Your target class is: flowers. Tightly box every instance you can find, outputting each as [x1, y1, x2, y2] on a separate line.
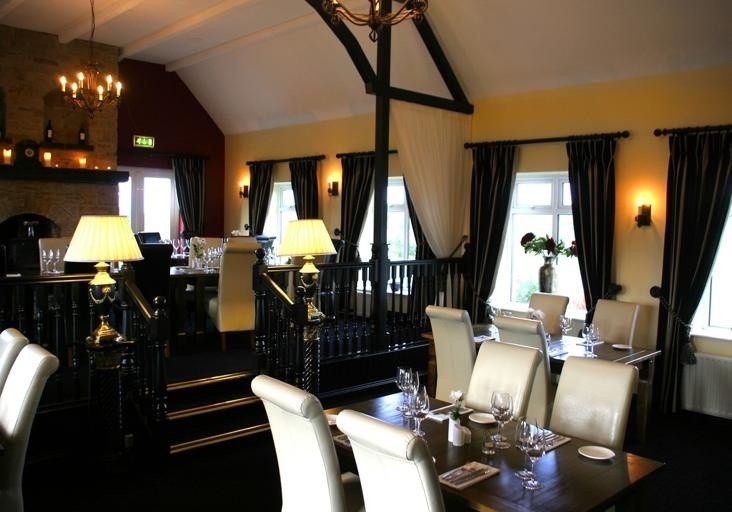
[520, 233, 577, 258]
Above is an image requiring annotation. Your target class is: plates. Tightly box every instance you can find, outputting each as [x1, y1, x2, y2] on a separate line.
[577, 445, 615, 461]
[612, 343, 633, 350]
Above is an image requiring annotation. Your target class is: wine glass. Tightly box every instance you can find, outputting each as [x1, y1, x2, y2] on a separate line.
[396, 367, 545, 490]
[582, 322, 595, 355]
[41, 249, 52, 273]
[559, 315, 574, 336]
[158, 239, 190, 258]
[50, 248, 60, 273]
[203, 245, 222, 273]
[588, 327, 599, 358]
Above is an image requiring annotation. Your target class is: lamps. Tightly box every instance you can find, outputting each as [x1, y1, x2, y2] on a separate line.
[55, 0, 126, 119]
[62, 215, 146, 346]
[634, 194, 652, 227]
[325, 173, 340, 196]
[277, 219, 337, 318]
[238, 176, 250, 199]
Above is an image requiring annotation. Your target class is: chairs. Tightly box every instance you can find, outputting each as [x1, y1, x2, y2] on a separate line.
[0, 342, 60, 512]
[135, 232, 262, 358]
[0, 328, 30, 392]
[40, 237, 71, 275]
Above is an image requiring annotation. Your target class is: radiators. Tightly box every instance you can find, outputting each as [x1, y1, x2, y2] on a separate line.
[681, 353, 732, 420]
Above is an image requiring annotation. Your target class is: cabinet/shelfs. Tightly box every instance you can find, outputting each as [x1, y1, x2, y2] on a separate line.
[0, 140, 130, 184]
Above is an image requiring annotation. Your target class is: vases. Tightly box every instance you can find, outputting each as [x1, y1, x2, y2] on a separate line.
[539, 257, 553, 293]
